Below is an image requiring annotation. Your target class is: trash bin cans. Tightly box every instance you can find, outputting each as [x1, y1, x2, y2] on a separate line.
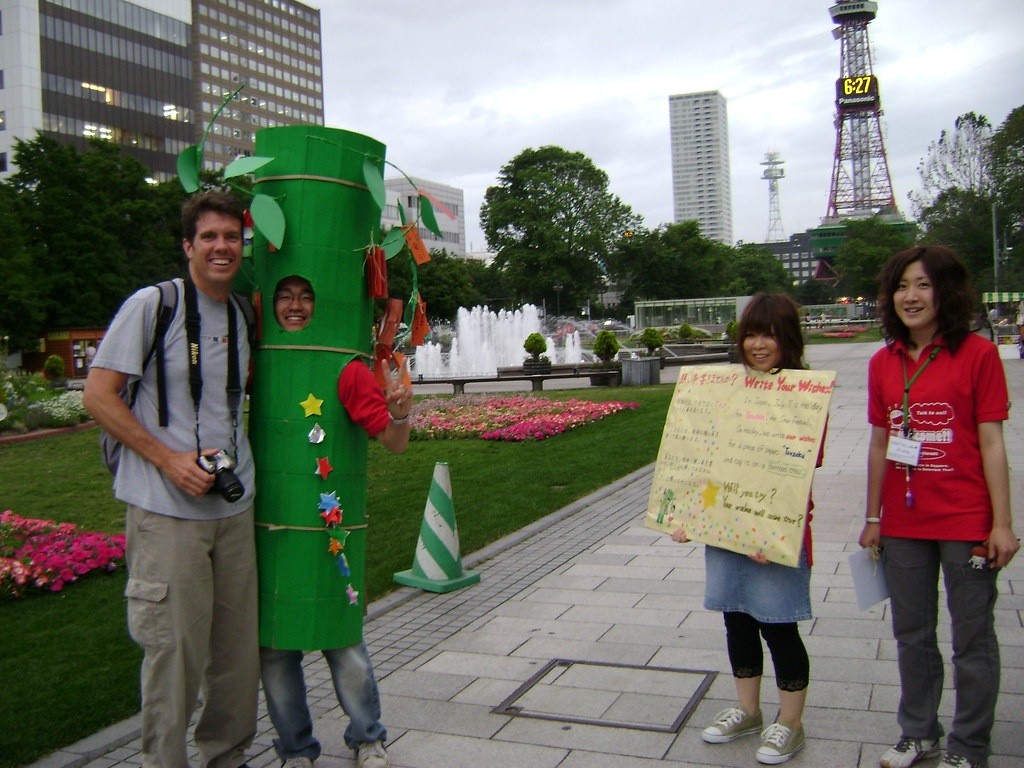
[640, 357, 661, 384]
[620, 356, 651, 387]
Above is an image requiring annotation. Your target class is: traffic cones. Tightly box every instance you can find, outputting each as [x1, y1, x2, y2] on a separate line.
[393, 461, 481, 594]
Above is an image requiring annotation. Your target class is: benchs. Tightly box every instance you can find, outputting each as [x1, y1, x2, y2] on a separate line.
[635, 339, 724, 348]
[411, 371, 619, 394]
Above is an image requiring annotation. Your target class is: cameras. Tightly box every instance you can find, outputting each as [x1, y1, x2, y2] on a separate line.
[195, 449, 244, 503]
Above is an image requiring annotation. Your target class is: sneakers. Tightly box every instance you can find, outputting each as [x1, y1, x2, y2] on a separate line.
[354, 738, 390, 768]
[880, 733, 942, 768]
[282, 756, 314, 767]
[702, 704, 764, 743]
[756, 716, 806, 765]
[937, 750, 988, 768]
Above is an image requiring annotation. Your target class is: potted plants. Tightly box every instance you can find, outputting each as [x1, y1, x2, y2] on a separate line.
[676, 323, 695, 344]
[590, 330, 622, 387]
[522, 333, 551, 375]
[641, 327, 666, 370]
[725, 319, 741, 364]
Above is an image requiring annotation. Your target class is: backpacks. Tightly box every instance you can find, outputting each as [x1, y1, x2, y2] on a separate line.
[99, 279, 256, 480]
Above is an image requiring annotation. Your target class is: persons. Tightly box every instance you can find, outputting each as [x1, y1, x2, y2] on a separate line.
[82, 190, 261, 768]
[805, 311, 826, 330]
[73, 342, 97, 372]
[243, 277, 412, 768]
[987, 305, 997, 322]
[672, 293, 830, 764]
[858, 247, 1020, 768]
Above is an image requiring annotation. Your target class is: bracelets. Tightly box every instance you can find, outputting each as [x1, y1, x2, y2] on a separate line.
[866, 517, 881, 522]
[388, 413, 410, 424]
